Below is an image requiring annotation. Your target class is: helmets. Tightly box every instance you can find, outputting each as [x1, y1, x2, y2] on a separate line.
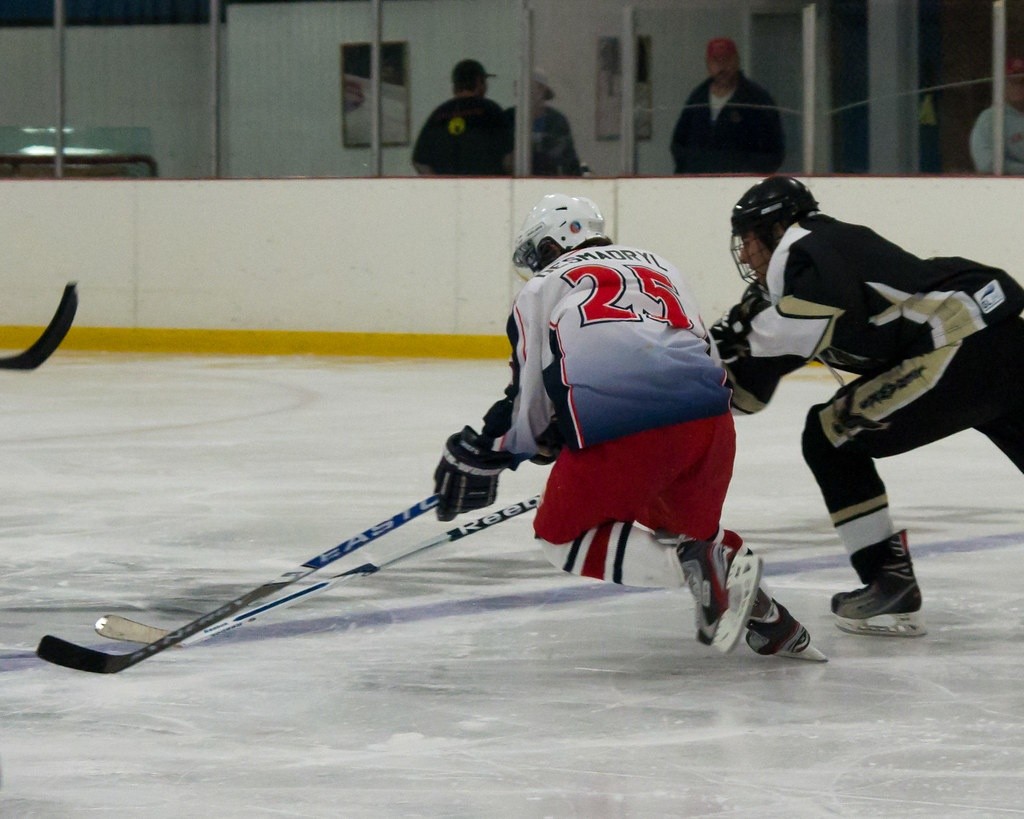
[508, 189, 615, 281]
[727, 175, 817, 296]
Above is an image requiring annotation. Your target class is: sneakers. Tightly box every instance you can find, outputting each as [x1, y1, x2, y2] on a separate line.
[833, 562, 926, 640]
[673, 540, 760, 652]
[741, 601, 831, 668]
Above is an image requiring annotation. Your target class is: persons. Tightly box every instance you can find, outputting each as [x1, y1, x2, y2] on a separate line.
[667, 36, 786, 172]
[502, 68, 587, 177]
[708, 170, 1022, 642]
[412, 57, 516, 174]
[429, 191, 826, 662]
[969, 58, 1023, 174]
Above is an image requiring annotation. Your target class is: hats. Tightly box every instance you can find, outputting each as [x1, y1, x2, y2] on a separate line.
[451, 61, 497, 83]
[1003, 57, 1024, 82]
[516, 64, 555, 101]
[704, 36, 744, 61]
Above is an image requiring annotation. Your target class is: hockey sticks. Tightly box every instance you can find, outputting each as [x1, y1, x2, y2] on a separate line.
[34, 493, 439, 675]
[94, 492, 541, 647]
[0, 280, 83, 372]
